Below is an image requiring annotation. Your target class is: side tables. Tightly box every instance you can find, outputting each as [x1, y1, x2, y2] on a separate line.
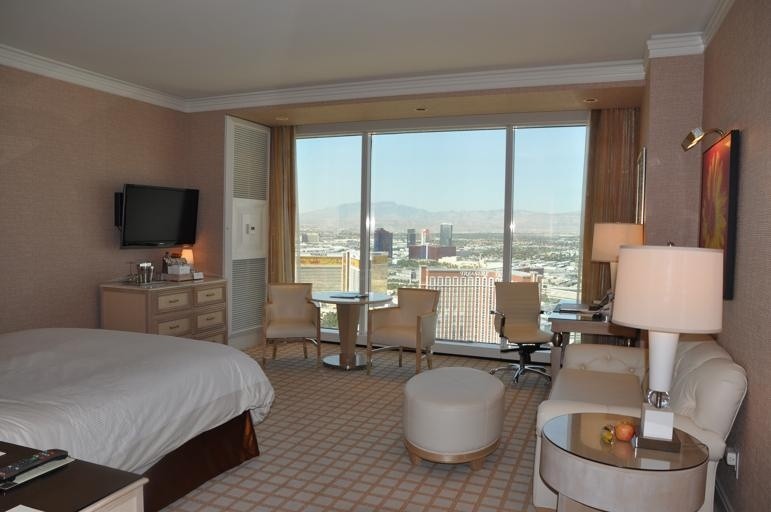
[540, 412, 709, 512]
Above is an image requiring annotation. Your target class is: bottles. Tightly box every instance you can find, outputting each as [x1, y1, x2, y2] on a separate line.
[162, 251, 170, 260]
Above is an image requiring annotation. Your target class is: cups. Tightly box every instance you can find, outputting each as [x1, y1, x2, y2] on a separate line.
[137, 265, 154, 283]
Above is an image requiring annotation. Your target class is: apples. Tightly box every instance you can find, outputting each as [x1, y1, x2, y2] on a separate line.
[615, 422, 634, 442]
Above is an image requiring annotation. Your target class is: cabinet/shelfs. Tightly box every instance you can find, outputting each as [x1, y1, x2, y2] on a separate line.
[98, 279, 230, 346]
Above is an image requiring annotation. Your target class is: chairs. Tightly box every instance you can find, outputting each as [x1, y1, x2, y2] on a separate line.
[262, 282, 321, 369]
[489, 281, 553, 387]
[367, 288, 441, 377]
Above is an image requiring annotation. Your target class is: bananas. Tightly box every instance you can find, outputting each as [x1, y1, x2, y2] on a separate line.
[601, 425, 613, 441]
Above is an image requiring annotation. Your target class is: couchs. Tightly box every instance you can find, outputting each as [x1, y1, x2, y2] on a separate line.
[532, 334, 748, 512]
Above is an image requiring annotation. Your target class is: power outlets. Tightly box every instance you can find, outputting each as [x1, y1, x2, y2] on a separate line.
[725, 446, 740, 480]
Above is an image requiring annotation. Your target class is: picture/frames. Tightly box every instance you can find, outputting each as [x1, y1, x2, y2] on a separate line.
[635, 146, 646, 224]
[699, 129, 740, 301]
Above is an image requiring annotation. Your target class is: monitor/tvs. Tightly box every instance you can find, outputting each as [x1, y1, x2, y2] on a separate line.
[120, 184, 199, 249]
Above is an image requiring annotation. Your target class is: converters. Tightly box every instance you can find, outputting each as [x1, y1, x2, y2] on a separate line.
[592, 312, 602, 319]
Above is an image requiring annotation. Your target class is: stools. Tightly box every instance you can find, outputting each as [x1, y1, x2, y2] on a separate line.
[402, 366, 506, 471]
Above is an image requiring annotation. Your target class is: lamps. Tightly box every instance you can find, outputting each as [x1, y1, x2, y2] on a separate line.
[591, 221, 644, 296]
[610, 246, 724, 442]
[680, 126, 725, 152]
[180, 249, 194, 265]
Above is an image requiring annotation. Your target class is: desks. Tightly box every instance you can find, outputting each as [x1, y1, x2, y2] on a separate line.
[303, 291, 394, 371]
[548, 298, 637, 387]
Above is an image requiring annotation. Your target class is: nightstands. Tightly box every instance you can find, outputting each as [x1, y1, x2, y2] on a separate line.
[1, 441, 151, 512]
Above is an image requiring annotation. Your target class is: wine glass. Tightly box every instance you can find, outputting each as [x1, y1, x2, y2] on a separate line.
[128, 262, 138, 281]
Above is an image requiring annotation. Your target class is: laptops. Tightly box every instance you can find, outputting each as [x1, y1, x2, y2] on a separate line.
[560, 303, 589, 311]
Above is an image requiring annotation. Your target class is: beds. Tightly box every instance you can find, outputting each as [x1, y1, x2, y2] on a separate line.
[0, 326, 259, 512]
